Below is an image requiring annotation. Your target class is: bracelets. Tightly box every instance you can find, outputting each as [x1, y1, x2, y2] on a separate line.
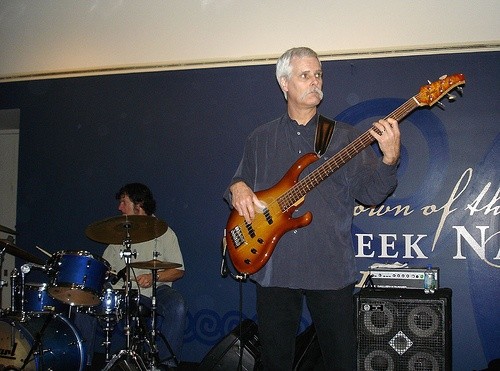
[156, 272, 160, 282]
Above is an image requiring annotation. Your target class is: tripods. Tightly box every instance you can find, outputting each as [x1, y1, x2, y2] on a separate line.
[103, 231, 180, 371]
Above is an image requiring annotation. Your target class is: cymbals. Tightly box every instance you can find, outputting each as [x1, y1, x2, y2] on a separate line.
[84, 216, 168, 245]
[0, 239, 35, 260]
[129, 260, 182, 269]
[0, 224, 24, 237]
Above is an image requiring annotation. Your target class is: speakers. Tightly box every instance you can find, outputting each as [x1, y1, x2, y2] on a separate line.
[351, 286, 453, 371]
[197, 317, 263, 371]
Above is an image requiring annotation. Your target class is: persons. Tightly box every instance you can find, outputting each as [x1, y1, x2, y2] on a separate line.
[222, 47, 400, 371]
[73, 182, 185, 371]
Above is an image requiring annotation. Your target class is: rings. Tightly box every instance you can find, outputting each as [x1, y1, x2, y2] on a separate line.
[381, 130, 385, 133]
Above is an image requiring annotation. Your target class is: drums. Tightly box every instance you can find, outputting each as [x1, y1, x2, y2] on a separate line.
[14, 264, 77, 323]
[0, 311, 86, 371]
[47, 250, 111, 308]
[85, 288, 139, 319]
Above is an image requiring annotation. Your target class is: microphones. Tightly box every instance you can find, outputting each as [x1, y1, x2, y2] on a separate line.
[112, 267, 126, 285]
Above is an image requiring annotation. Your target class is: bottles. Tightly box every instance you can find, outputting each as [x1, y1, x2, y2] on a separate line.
[424, 264, 435, 294]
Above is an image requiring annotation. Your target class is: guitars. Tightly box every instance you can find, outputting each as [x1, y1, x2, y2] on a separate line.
[226, 72, 465, 276]
